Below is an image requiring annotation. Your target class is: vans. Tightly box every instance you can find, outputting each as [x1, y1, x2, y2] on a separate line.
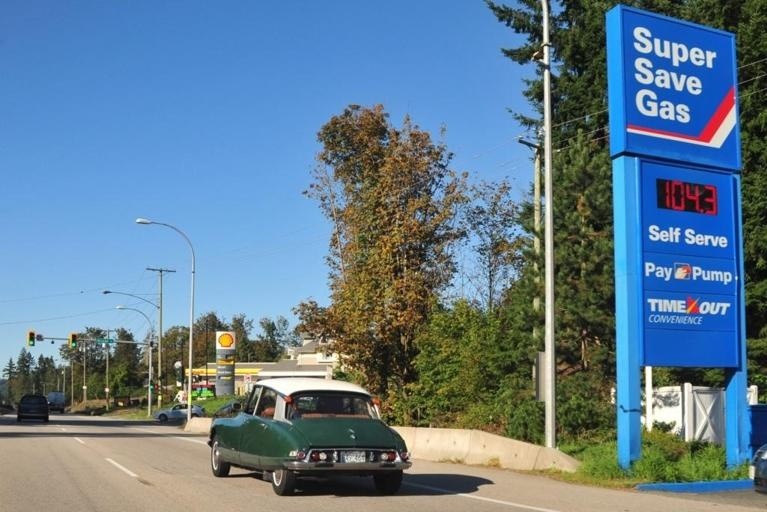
[15, 392, 50, 423]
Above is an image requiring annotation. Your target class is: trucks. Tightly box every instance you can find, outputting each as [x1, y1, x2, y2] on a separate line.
[47, 390, 66, 415]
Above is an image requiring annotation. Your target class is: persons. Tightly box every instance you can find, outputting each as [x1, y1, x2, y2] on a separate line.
[260, 404, 301, 419]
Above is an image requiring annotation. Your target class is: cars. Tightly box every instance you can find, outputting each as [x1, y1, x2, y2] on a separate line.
[746, 442, 766, 496]
[155, 400, 207, 424]
[204, 374, 414, 497]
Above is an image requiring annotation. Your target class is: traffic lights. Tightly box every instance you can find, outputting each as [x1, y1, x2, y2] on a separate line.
[26, 330, 36, 347]
[70, 333, 77, 349]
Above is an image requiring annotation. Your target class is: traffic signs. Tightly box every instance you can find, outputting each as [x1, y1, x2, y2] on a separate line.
[96, 338, 114, 344]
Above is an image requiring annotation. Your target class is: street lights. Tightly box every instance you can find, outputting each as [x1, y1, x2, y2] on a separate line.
[102, 327, 114, 412]
[114, 304, 154, 418]
[101, 289, 164, 409]
[178, 326, 185, 390]
[133, 216, 196, 420]
